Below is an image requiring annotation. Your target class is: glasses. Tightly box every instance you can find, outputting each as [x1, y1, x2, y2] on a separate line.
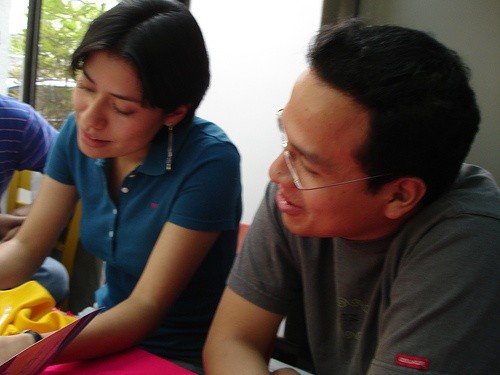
[275, 108, 388, 192]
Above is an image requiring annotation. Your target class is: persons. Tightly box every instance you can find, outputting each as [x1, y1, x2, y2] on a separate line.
[203, 18, 499, 374]
[1, 95, 71, 309]
[0, 0, 242, 375]
